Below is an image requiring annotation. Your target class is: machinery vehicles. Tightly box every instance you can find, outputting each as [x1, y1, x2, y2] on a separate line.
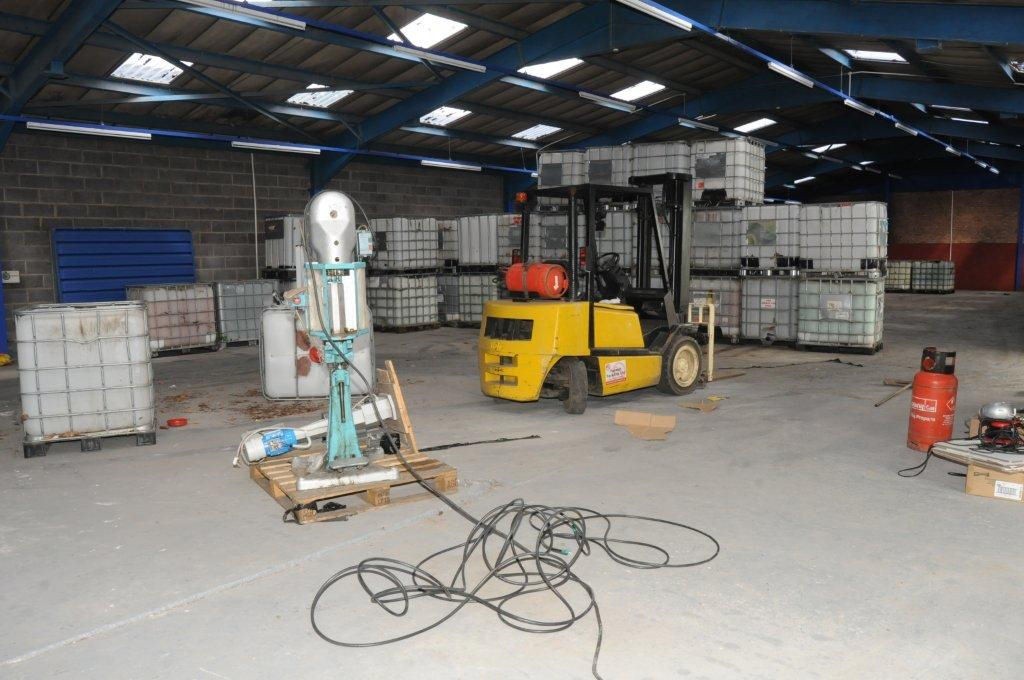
[478, 171, 749, 415]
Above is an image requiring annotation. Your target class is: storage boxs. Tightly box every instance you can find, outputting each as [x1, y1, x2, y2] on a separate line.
[15, 129, 954, 456]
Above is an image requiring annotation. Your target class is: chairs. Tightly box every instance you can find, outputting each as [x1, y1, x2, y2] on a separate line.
[546, 258, 587, 302]
